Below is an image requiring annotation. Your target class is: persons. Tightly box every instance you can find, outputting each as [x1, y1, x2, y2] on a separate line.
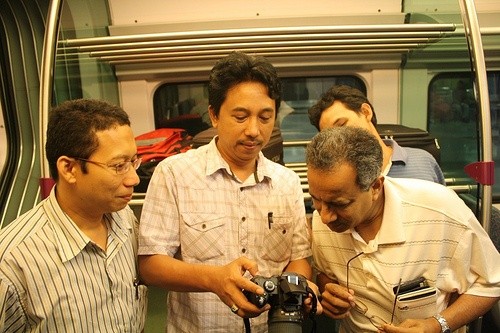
[306, 83, 446, 186]
[0, 96, 150, 333]
[309, 125, 500, 333]
[152, 80, 500, 132]
[134, 50, 312, 333]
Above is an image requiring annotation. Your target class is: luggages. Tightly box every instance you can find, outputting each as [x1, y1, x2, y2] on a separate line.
[193, 126, 284, 166]
[374, 123, 440, 165]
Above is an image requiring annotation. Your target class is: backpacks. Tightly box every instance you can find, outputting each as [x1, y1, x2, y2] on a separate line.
[134, 128, 192, 181]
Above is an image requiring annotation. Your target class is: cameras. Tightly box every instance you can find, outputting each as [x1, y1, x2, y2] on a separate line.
[242, 272, 309, 333]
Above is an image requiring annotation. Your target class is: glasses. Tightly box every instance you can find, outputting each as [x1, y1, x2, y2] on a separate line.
[69, 155, 142, 176]
[344, 252, 403, 332]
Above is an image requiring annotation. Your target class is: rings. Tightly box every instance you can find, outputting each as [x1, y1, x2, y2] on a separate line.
[231, 303, 238, 312]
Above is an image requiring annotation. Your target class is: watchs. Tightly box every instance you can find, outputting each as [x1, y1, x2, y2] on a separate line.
[433, 313, 452, 333]
